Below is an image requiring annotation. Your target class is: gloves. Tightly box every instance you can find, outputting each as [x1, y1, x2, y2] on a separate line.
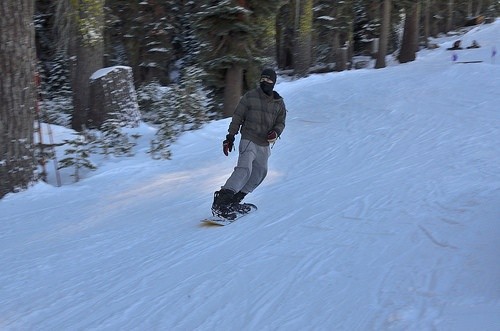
[222, 134, 235, 156]
[267, 130, 278, 142]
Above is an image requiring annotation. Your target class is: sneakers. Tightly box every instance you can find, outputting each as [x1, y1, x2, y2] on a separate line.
[228, 202, 253, 214]
[211, 204, 237, 221]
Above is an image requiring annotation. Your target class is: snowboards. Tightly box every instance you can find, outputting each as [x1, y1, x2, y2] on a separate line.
[200, 202, 257, 225]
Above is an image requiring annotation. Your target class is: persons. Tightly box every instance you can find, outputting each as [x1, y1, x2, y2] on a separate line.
[211, 68, 287, 222]
[454, 39, 479, 48]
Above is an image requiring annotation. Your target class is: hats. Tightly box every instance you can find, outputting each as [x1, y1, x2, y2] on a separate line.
[260, 69, 277, 82]
